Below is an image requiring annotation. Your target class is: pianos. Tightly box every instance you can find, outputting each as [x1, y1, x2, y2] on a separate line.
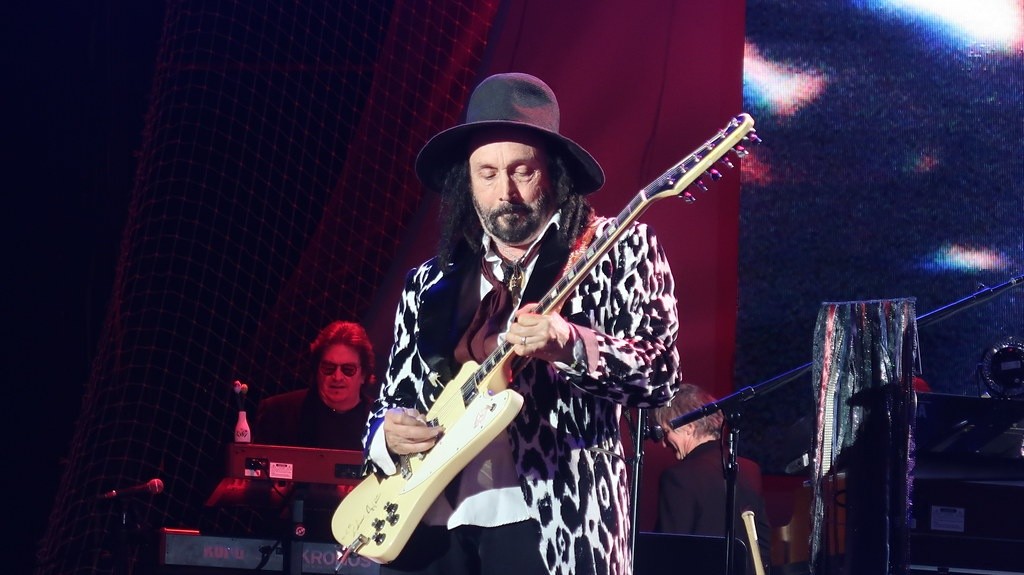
[158, 526, 380, 574]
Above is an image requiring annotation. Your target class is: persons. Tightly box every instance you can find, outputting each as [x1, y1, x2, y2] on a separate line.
[360, 73, 683, 575]
[654, 382, 772, 575]
[250, 322, 377, 539]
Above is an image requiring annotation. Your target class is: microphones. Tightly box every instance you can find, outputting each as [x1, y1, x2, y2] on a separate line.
[99, 479, 164, 499]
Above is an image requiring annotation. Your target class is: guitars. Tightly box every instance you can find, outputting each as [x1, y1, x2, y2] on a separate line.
[331, 110, 762, 566]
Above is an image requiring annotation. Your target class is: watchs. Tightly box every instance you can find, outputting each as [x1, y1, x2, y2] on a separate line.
[567, 334, 586, 371]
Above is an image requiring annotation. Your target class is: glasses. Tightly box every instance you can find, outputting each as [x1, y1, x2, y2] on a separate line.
[320, 357, 361, 376]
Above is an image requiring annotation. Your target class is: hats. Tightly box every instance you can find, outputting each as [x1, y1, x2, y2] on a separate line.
[416, 72, 605, 199]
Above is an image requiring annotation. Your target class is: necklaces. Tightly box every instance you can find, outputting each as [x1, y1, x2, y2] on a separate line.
[498, 254, 527, 297]
